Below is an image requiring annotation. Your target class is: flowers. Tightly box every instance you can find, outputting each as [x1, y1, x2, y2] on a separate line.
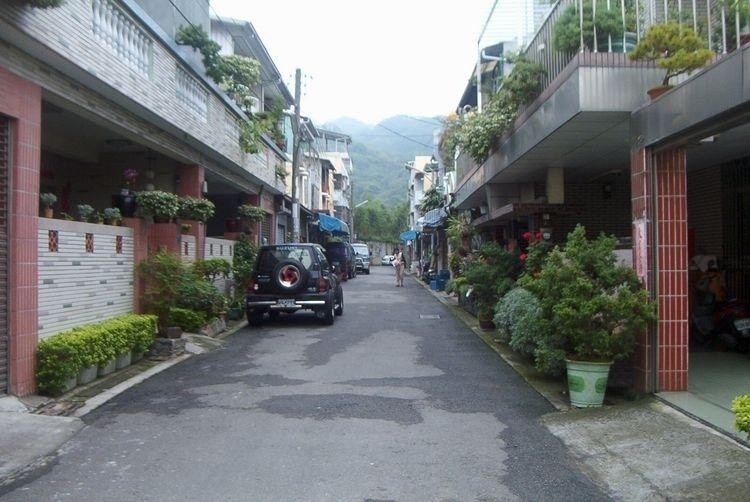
[122, 167, 139, 196]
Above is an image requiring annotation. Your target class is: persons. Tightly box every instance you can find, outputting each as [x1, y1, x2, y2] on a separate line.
[392, 247, 406, 287]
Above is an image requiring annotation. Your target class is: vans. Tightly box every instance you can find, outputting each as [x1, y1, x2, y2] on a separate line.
[318, 241, 373, 281]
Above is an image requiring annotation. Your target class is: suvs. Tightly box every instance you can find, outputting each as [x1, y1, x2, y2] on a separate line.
[246, 242, 343, 328]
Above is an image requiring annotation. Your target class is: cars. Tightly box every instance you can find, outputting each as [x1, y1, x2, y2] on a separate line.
[381, 254, 394, 266]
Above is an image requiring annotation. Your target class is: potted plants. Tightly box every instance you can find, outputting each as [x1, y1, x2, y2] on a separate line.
[210, 295, 245, 324]
[477, 300, 491, 331]
[629, 20, 716, 100]
[35, 313, 160, 398]
[40, 192, 120, 225]
[133, 188, 179, 224]
[532, 222, 660, 407]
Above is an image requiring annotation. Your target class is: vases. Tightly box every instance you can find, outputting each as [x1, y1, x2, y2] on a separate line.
[122, 194, 135, 218]
